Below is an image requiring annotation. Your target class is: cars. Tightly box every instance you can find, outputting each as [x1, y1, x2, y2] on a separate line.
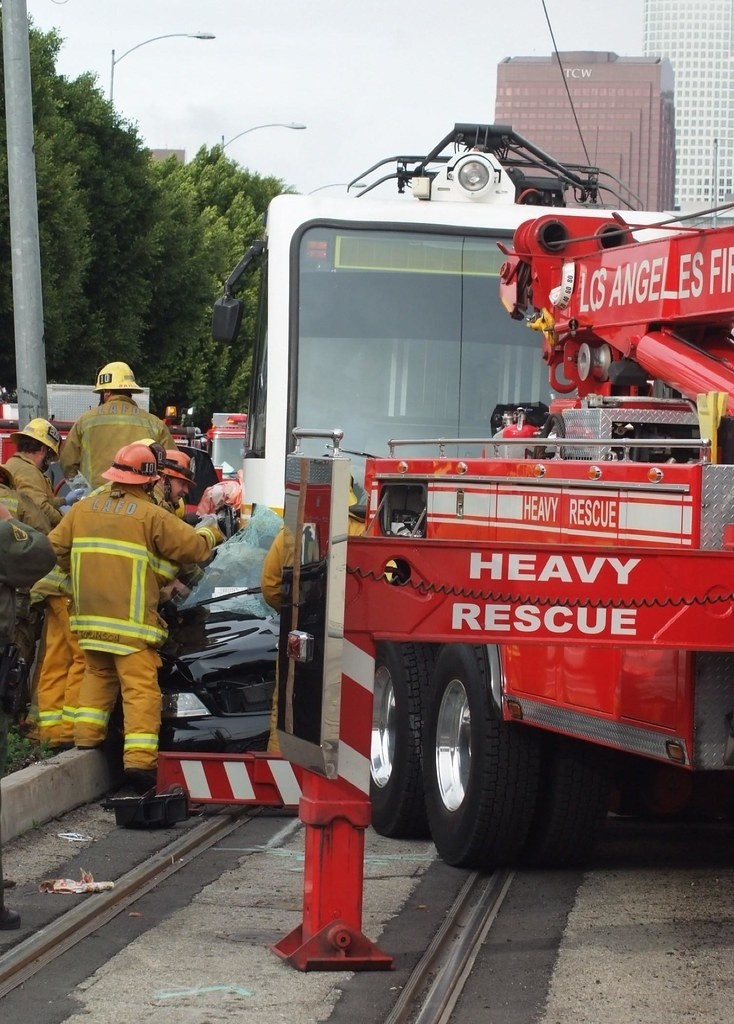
[159, 501, 284, 750]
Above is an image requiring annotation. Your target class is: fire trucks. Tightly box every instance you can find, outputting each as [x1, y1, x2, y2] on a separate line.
[154, 216, 734, 975]
[0, 379, 250, 490]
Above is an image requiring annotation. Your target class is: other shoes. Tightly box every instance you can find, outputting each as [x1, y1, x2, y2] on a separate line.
[126, 765, 157, 794]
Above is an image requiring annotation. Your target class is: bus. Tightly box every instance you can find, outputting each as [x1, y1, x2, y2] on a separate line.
[207, 119, 734, 553]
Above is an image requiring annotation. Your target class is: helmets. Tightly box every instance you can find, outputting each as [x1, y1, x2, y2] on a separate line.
[10, 417, 60, 462]
[101, 438, 166, 484]
[92, 362, 144, 394]
[0, 463, 14, 489]
[164, 449, 197, 489]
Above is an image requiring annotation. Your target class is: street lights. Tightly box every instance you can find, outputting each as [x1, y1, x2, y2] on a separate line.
[217, 124, 305, 156]
[109, 31, 216, 116]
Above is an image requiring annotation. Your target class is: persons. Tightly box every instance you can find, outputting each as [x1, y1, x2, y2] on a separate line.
[0, 503, 59, 930]
[49, 445, 221, 789]
[260, 484, 367, 754]
[1, 360, 207, 751]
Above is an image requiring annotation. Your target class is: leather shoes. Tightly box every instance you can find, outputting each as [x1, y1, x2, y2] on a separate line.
[0, 905, 21, 928]
